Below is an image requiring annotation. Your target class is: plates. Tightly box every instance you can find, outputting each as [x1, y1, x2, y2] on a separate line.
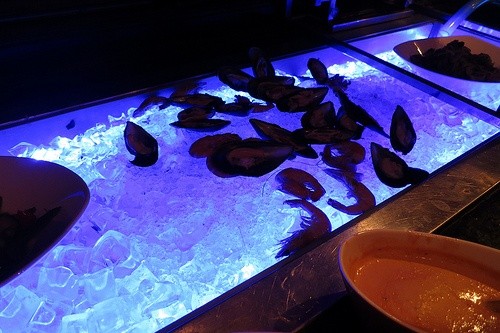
[1, 155, 90, 290]
[392, 34, 500, 91]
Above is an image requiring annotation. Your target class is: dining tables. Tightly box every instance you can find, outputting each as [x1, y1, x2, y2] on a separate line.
[2, 11, 498, 333]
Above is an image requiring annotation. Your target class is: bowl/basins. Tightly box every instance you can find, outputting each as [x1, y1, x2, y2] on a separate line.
[339, 229, 499, 333]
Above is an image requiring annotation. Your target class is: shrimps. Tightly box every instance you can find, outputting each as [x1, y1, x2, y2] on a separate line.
[275, 168, 326, 202]
[319, 141, 367, 166]
[272, 200, 332, 260]
[321, 169, 376, 216]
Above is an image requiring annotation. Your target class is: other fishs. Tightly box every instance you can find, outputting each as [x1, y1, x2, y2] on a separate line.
[370, 142, 430, 188]
[133, 95, 170, 117]
[122, 121, 158, 168]
[168, 59, 387, 178]
[391, 104, 416, 154]
